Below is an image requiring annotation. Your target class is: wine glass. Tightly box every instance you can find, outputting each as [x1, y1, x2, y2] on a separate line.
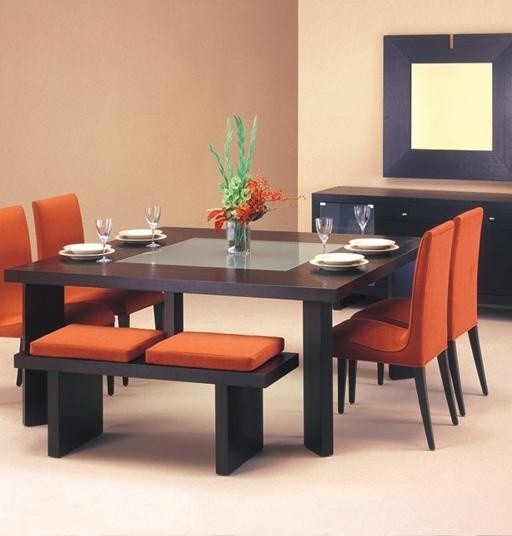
[353, 204, 371, 236]
[94, 217, 113, 264]
[314, 217, 334, 254]
[143, 203, 163, 248]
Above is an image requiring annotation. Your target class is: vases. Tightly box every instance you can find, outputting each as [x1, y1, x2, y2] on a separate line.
[226, 214, 250, 254]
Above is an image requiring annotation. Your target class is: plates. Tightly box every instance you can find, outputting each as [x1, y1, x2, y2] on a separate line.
[59, 241, 116, 262]
[307, 252, 369, 271]
[114, 228, 168, 243]
[343, 237, 400, 255]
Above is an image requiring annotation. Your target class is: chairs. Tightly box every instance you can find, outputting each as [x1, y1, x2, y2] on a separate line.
[331, 206, 490, 450]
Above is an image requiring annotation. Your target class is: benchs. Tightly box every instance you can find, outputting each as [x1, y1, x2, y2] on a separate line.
[13, 321, 299, 476]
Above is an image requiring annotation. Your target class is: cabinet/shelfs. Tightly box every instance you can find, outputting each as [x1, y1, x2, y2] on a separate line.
[311, 195, 511, 310]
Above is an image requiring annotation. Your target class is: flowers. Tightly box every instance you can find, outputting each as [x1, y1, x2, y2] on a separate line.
[205, 114, 309, 252]
